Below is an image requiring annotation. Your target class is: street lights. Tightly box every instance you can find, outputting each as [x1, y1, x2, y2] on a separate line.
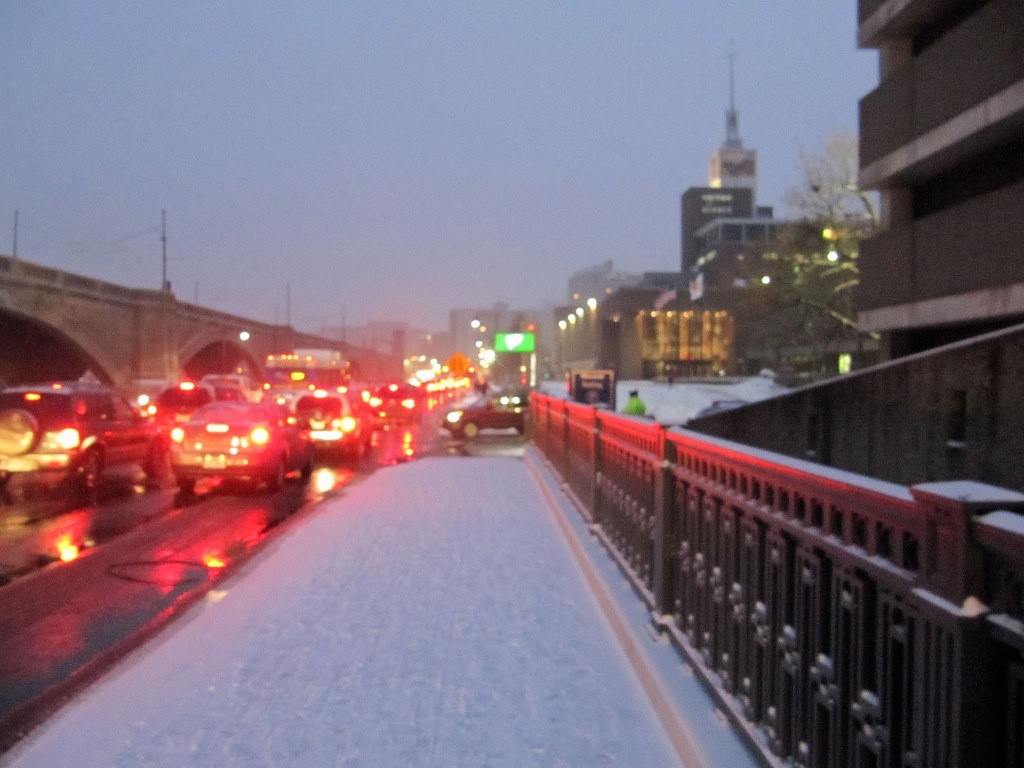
[576, 307, 586, 372]
[559, 320, 566, 381]
[567, 314, 576, 371]
[588, 298, 597, 368]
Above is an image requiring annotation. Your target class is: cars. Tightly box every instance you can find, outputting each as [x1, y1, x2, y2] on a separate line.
[203, 372, 269, 403]
[147, 380, 248, 425]
[289, 389, 369, 457]
[171, 401, 315, 489]
[370, 382, 424, 427]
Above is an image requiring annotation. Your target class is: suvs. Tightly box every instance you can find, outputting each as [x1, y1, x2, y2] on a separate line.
[437, 387, 547, 438]
[0, 381, 163, 503]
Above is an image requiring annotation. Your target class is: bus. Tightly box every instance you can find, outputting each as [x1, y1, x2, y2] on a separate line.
[264, 348, 360, 394]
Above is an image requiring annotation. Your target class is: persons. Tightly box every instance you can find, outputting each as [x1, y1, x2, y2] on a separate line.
[624, 391, 646, 415]
